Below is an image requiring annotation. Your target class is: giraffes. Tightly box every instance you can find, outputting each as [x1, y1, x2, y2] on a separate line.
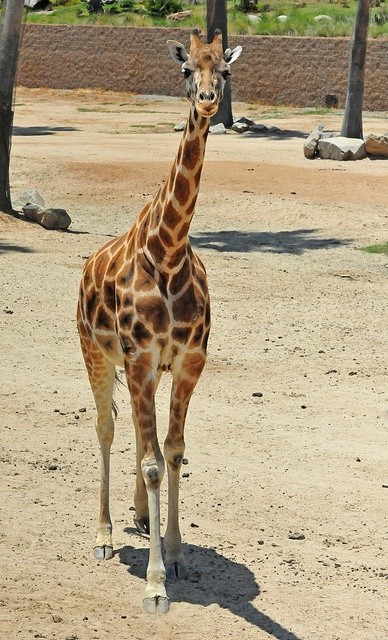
[76, 28, 244, 614]
